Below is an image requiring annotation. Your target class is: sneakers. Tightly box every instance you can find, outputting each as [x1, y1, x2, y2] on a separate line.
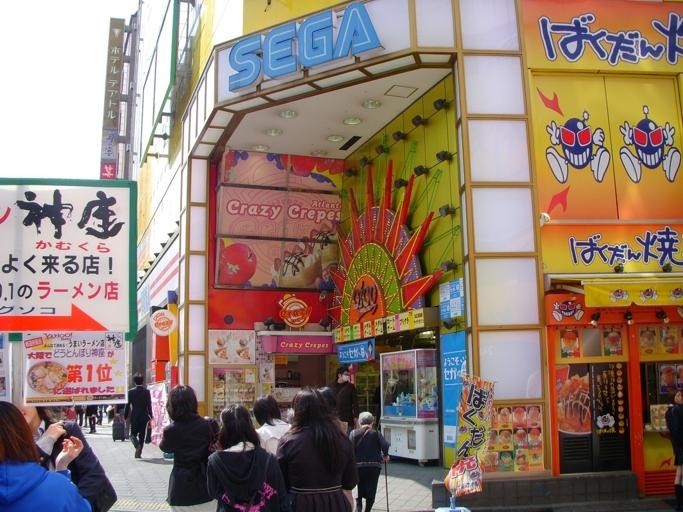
[135, 446, 142, 459]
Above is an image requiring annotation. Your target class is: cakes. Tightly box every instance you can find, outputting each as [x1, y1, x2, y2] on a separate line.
[270, 224, 340, 290]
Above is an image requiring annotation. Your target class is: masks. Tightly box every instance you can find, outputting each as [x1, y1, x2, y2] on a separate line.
[342, 375, 348, 382]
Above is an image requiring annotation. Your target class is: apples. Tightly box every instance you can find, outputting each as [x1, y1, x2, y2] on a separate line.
[224, 244, 257, 284]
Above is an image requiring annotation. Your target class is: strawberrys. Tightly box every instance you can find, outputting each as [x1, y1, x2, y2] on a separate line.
[219, 239, 240, 285]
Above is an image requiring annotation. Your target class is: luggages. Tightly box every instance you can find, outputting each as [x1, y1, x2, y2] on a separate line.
[112, 418, 125, 442]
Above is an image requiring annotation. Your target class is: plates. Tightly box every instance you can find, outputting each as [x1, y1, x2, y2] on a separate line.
[557, 427, 592, 438]
[27, 362, 68, 394]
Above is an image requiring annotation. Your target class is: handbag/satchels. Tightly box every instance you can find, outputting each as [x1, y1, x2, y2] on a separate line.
[145, 426, 152, 444]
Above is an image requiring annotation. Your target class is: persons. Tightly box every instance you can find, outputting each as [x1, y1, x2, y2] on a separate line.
[124, 372, 153, 458]
[0, 401, 92, 512]
[16, 406, 117, 512]
[158, 363, 390, 512]
[65, 394, 131, 441]
